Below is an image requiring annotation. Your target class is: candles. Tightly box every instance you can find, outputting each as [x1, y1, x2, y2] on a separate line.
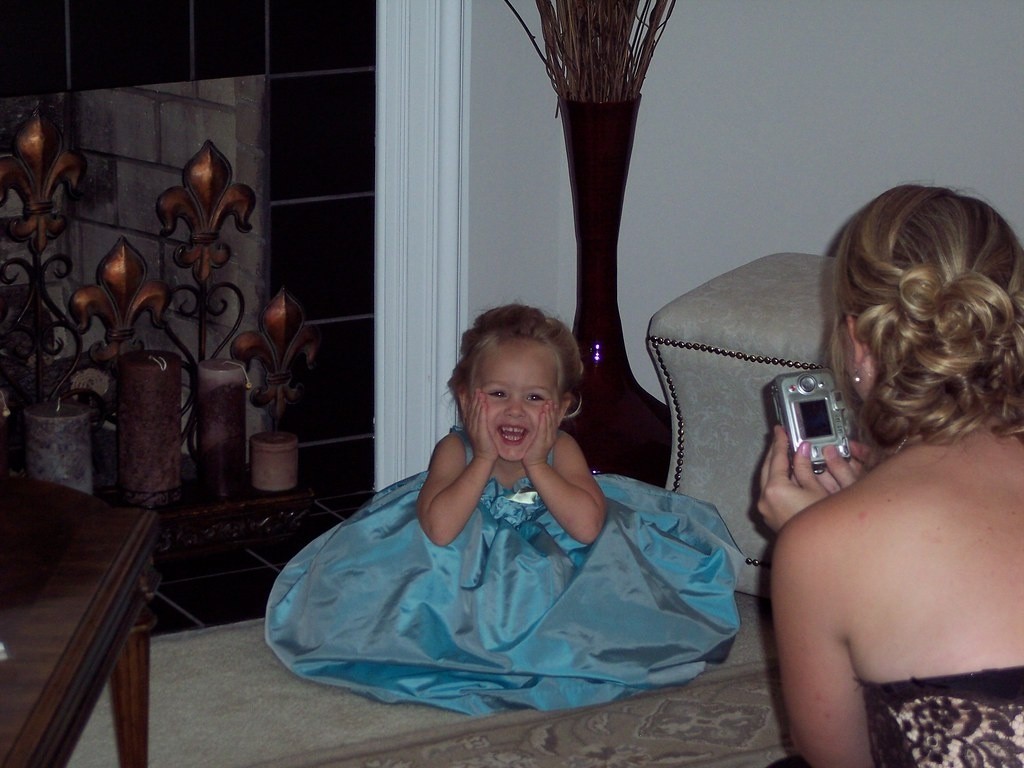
[198, 359, 252, 497]
[117, 349, 183, 507]
[249, 431, 298, 491]
[24, 396, 94, 496]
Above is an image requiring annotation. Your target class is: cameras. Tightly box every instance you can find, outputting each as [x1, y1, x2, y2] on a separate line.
[770, 368, 853, 473]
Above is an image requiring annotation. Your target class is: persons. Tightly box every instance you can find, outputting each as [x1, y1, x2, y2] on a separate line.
[265, 303, 750, 718]
[756, 184, 1024, 768]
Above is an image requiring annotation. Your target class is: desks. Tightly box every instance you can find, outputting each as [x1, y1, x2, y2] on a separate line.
[0, 492, 172, 768]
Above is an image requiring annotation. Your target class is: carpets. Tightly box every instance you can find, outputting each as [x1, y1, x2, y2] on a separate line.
[250, 656, 796, 768]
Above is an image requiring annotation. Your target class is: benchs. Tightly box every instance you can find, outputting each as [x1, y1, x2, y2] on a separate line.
[647, 252, 834, 597]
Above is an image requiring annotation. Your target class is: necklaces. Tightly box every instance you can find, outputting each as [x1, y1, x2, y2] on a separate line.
[892, 435, 909, 454]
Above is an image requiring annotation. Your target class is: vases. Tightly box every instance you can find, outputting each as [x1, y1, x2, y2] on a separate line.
[558, 93, 672, 489]
